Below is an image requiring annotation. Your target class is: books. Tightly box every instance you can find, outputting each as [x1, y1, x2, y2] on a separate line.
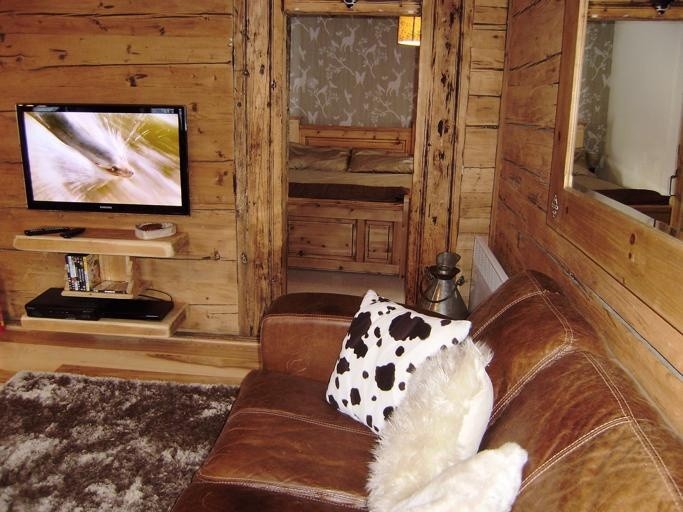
[65, 253, 134, 294]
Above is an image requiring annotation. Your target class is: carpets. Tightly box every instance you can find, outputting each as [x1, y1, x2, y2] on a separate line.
[1, 368, 238, 511]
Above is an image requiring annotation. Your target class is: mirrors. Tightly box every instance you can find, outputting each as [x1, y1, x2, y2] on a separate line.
[546, 2, 681, 332]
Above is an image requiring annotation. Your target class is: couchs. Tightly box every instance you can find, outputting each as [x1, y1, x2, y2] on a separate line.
[168, 270, 682, 512]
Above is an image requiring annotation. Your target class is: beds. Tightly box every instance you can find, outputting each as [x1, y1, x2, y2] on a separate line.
[281, 125, 416, 281]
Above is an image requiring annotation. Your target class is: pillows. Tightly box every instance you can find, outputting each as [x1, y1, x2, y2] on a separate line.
[284, 141, 412, 174]
[364, 339, 495, 511]
[383, 441, 528, 507]
[325, 287, 473, 439]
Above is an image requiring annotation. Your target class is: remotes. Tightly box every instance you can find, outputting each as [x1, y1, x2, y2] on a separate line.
[24, 226, 70, 235]
[63, 228, 85, 238]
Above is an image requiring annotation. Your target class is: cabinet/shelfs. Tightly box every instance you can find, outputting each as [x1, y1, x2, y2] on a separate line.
[10, 230, 187, 339]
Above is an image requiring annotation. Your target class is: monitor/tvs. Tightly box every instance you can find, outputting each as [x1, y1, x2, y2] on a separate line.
[17, 103, 190, 215]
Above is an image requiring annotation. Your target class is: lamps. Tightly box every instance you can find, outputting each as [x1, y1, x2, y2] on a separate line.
[396, 6, 421, 48]
